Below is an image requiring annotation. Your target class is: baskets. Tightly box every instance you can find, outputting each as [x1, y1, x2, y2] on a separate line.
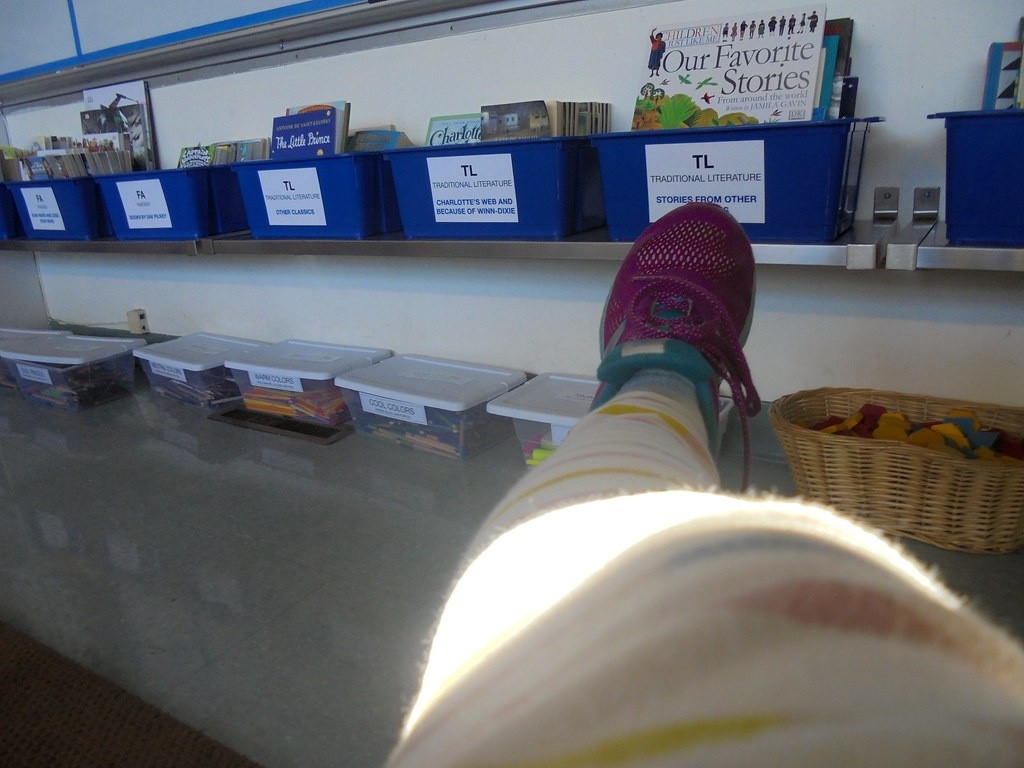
[767, 387, 1024, 556]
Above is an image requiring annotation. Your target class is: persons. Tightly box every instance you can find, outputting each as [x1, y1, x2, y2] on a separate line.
[387, 200, 1023, 768]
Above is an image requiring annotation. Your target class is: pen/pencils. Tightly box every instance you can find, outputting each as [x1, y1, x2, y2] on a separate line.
[363, 411, 473, 458]
[21, 378, 126, 405]
[153, 377, 242, 407]
[242, 386, 347, 424]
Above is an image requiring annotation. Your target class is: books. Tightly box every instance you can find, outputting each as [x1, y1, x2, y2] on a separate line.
[0, 2, 1024, 182]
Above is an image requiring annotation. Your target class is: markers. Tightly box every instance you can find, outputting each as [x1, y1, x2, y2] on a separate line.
[522, 430, 554, 464]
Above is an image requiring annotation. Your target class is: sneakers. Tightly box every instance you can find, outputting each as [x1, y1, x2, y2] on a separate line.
[591, 202, 763, 492]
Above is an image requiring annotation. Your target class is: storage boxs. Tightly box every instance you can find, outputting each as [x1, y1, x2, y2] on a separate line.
[230, 149, 403, 240]
[0, 175, 116, 240]
[0, 326, 147, 412]
[588, 118, 885, 243]
[927, 108, 1024, 248]
[382, 134, 606, 240]
[335, 353, 528, 462]
[132, 332, 274, 417]
[90, 163, 251, 240]
[486, 372, 734, 471]
[225, 338, 393, 426]
